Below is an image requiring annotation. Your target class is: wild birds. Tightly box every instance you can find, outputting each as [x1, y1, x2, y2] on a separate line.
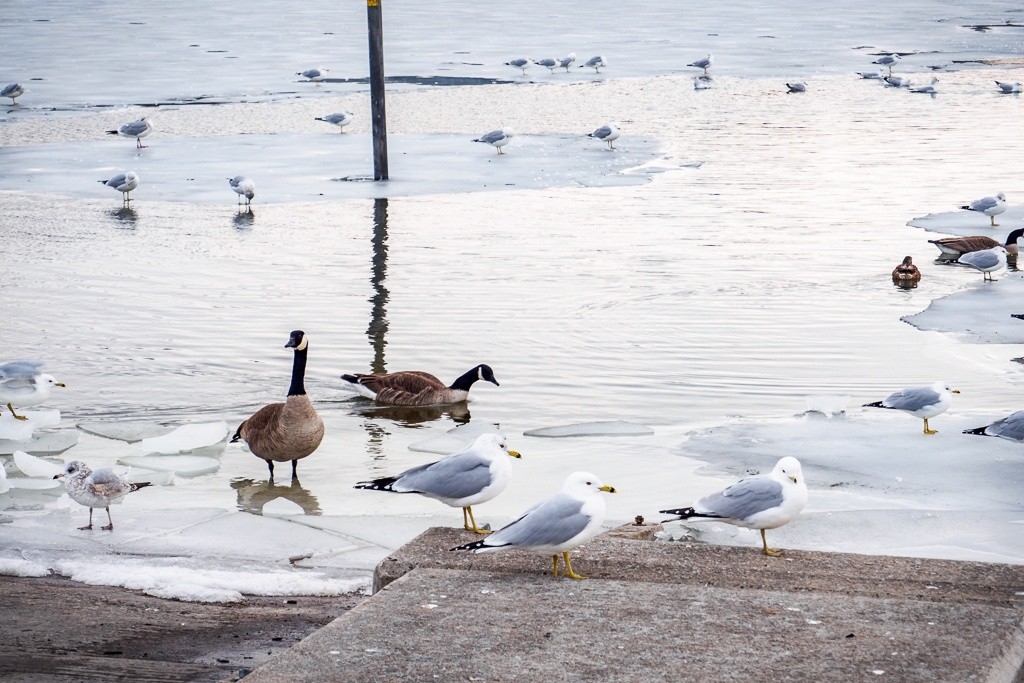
[928, 227, 1024, 282]
[994, 80, 1023, 93]
[960, 191, 1007, 226]
[0, 51, 808, 205]
[346, 407, 471, 427]
[111, 206, 138, 229]
[230, 478, 323, 516]
[353, 433, 524, 533]
[52, 461, 154, 530]
[232, 206, 256, 233]
[447, 472, 618, 578]
[340, 365, 500, 406]
[861, 381, 1023, 445]
[0, 361, 66, 421]
[230, 329, 326, 478]
[856, 53, 940, 93]
[891, 256, 921, 289]
[657, 456, 806, 557]
